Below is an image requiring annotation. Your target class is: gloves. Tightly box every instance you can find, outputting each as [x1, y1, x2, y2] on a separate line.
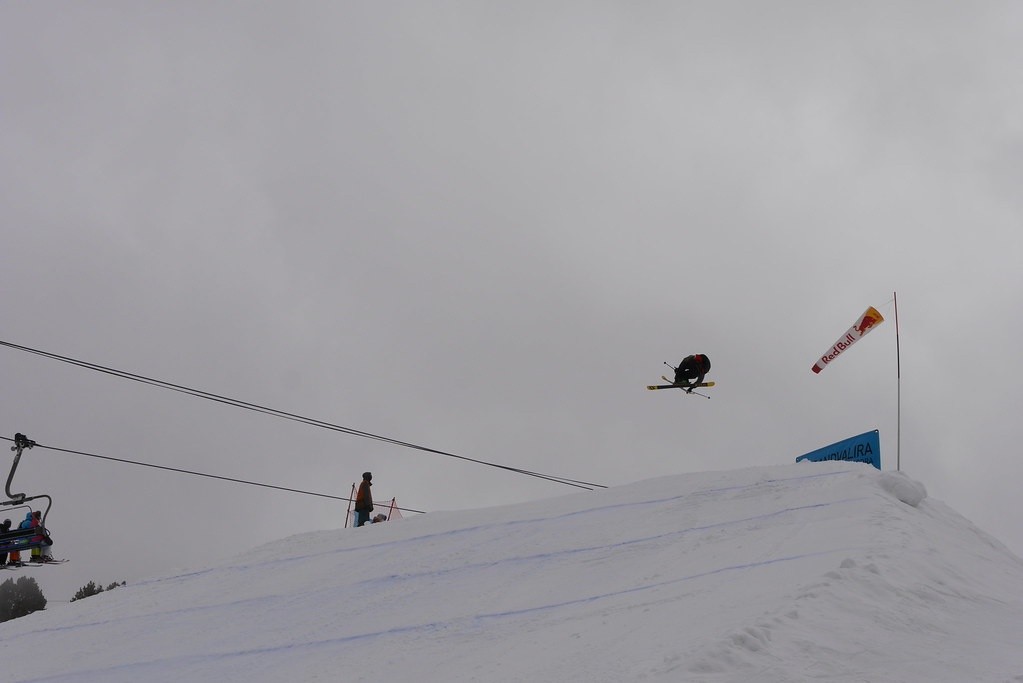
[369, 508, 373, 512]
[687, 388, 692, 393]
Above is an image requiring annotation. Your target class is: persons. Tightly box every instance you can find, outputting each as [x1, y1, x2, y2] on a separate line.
[0, 511, 53, 570]
[672, 354, 711, 394]
[372, 514, 387, 523]
[355, 472, 374, 526]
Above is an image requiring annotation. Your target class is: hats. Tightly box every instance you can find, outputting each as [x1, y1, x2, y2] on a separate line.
[362, 472, 372, 480]
[4, 518, 11, 526]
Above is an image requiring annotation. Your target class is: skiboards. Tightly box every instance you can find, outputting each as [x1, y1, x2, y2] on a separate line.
[0, 564, 43, 570]
[23, 558, 70, 565]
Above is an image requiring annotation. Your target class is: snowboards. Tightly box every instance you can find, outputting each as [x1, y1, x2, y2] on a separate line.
[647, 381, 716, 391]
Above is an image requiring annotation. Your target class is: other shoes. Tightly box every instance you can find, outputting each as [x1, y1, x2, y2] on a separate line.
[30, 557, 43, 563]
[43, 556, 52, 561]
[9, 559, 25, 566]
